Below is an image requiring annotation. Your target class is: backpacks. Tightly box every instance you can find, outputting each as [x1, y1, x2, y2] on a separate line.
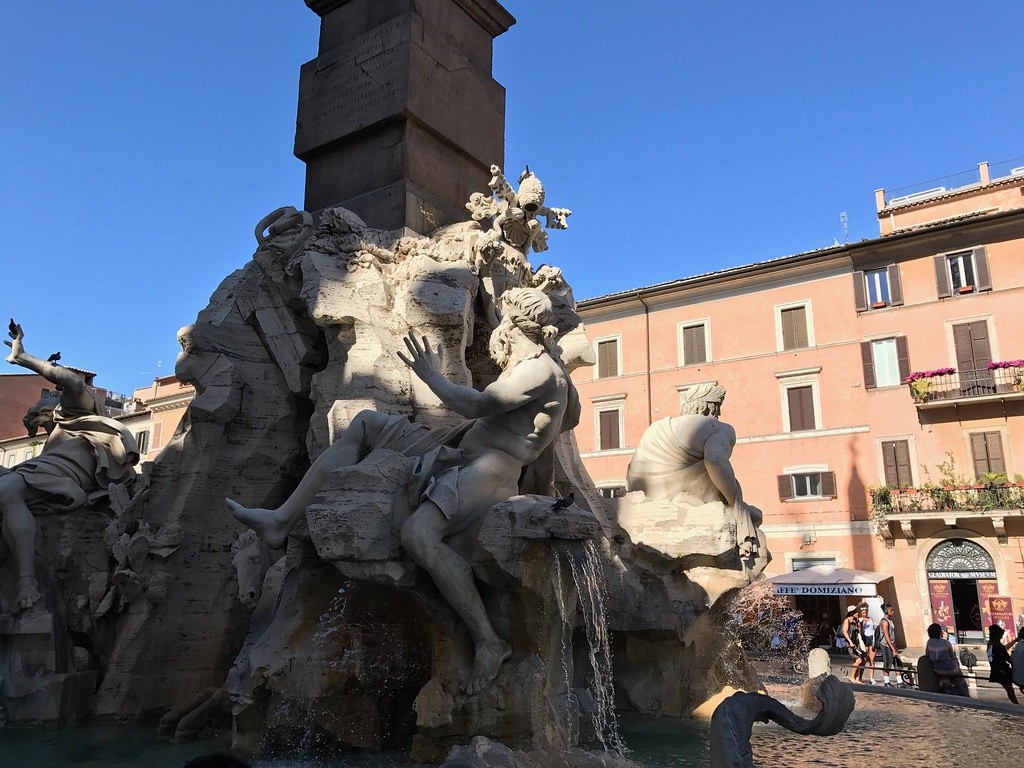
[875, 618, 890, 649]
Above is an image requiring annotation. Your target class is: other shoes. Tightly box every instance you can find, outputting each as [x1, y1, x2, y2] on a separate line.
[885, 682, 895, 688]
[869, 681, 877, 685]
[848, 677, 857, 684]
[897, 682, 909, 688]
[856, 680, 866, 684]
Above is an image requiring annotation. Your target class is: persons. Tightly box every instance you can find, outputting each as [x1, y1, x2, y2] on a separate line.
[628, 379, 773, 572]
[0, 317, 139, 615]
[873, 604, 908, 689]
[855, 601, 878, 686]
[842, 605, 868, 685]
[925, 623, 971, 698]
[986, 622, 1024, 705]
[770, 636, 790, 660]
[223, 288, 582, 695]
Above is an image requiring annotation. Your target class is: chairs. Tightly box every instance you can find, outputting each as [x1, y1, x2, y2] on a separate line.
[819, 635, 832, 660]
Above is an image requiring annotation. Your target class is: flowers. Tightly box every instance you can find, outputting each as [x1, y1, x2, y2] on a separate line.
[906, 367, 956, 383]
[988, 359, 1024, 371]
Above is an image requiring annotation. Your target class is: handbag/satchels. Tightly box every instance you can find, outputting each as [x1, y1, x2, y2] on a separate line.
[960, 647, 977, 674]
[837, 619, 850, 638]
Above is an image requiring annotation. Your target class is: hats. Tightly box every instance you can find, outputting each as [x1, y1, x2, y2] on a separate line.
[845, 605, 860, 617]
[989, 624, 1005, 638]
